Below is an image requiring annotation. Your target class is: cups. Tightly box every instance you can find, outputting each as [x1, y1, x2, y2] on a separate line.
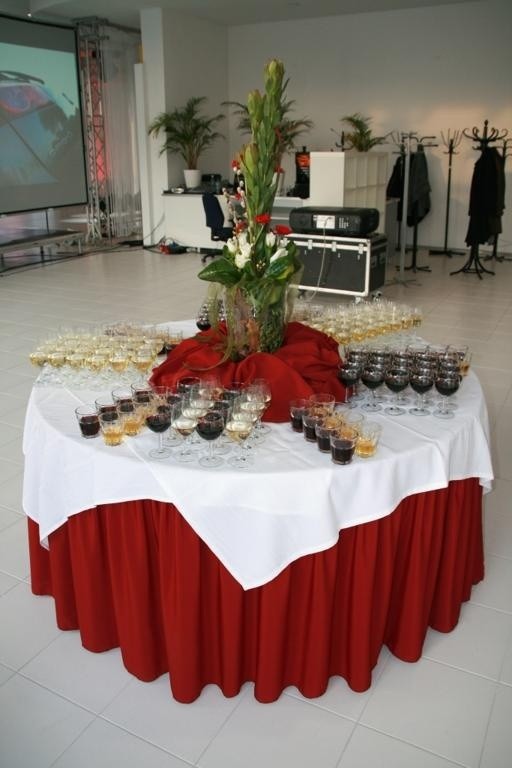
[195, 296, 226, 330]
[286, 391, 381, 467]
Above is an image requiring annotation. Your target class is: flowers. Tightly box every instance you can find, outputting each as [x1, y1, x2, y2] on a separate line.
[197, 54, 315, 353]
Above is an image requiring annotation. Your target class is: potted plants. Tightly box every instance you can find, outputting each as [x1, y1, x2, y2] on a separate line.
[148, 97, 227, 189]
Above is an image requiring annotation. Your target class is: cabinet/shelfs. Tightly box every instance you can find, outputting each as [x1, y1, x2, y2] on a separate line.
[307, 148, 390, 247]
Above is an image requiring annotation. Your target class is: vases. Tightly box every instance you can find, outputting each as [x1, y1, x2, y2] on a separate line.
[226, 288, 286, 351]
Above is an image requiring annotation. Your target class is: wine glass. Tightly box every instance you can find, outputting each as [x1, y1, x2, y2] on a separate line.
[28, 318, 272, 468]
[297, 296, 474, 421]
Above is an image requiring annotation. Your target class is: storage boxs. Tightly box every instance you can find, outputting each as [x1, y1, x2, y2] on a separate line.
[283, 233, 389, 297]
